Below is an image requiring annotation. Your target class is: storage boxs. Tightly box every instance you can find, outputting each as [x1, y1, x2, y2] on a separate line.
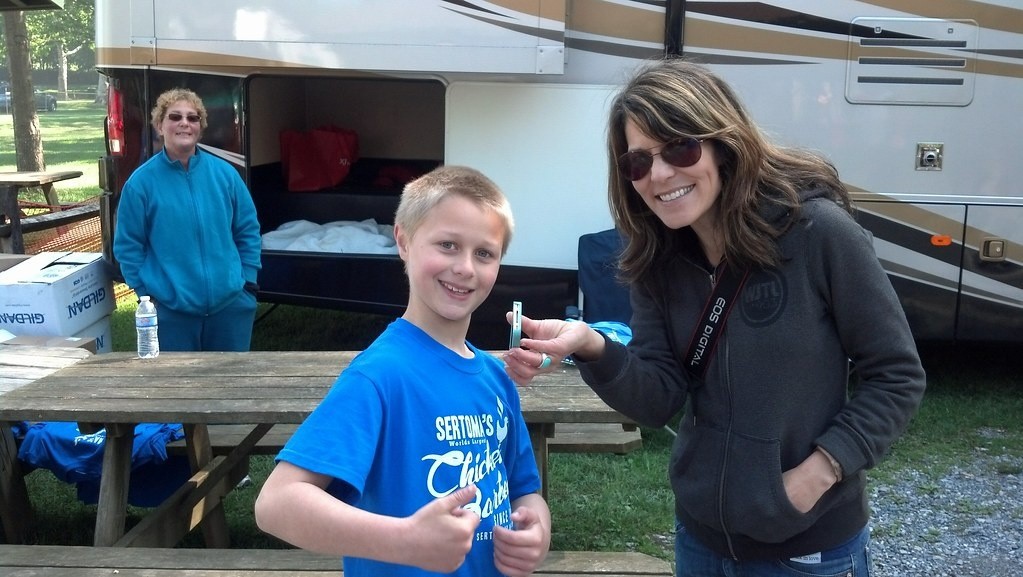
[0, 249, 118, 356]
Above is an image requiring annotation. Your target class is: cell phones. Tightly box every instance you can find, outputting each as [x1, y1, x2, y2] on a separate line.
[509, 301, 522, 349]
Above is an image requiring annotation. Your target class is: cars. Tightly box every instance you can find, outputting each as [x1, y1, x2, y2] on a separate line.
[0, 83, 60, 115]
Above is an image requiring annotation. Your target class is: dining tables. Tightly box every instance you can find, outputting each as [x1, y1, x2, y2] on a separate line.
[0, 170, 82, 222]
[0, 342, 645, 545]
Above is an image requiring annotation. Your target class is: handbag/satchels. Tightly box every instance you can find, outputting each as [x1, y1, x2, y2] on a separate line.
[280, 123, 358, 193]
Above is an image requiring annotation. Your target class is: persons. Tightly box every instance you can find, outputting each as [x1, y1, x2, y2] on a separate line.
[505, 62, 927, 576]
[112, 88, 262, 488]
[536, 352, 551, 369]
[255, 165, 551, 576]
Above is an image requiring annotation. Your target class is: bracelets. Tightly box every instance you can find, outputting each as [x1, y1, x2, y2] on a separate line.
[815, 444, 843, 483]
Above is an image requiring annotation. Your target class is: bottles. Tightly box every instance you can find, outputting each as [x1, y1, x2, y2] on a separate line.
[134, 295, 159, 358]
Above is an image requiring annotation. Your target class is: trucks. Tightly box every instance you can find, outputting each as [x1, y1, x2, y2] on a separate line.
[91, 0, 1023, 381]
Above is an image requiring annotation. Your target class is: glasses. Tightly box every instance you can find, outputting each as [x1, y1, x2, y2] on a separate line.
[617, 135, 712, 182]
[165, 114, 202, 122]
